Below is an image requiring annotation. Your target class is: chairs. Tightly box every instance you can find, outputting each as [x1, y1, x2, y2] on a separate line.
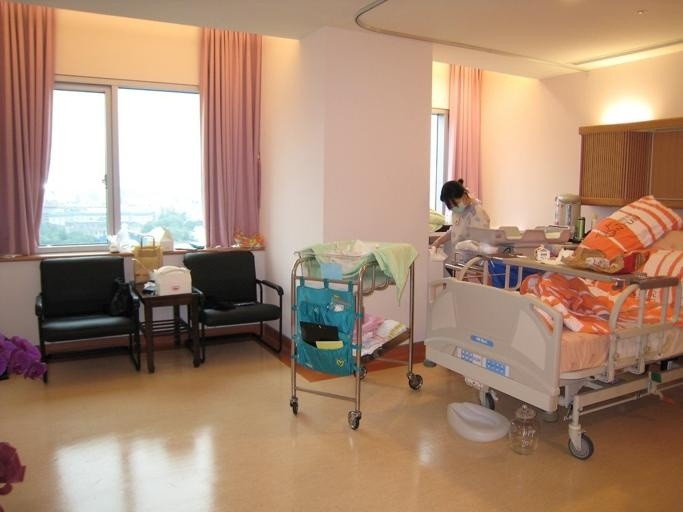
[182, 250, 284, 363]
[35, 255, 140, 384]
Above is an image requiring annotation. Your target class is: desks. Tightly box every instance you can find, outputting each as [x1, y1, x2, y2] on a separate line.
[128, 281, 201, 374]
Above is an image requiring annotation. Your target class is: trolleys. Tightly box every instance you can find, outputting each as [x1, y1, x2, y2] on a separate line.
[289, 249, 424, 430]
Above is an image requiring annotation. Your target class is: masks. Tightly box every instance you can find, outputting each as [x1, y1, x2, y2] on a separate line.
[451, 202, 466, 213]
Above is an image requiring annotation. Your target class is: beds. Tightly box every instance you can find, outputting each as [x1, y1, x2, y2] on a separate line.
[421, 256, 682, 460]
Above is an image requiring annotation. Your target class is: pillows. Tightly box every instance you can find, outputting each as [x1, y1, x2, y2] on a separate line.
[560, 194, 682, 274]
[621, 249, 682, 308]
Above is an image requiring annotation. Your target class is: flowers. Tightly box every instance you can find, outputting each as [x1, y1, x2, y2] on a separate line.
[0, 331, 47, 382]
[0, 441, 26, 496]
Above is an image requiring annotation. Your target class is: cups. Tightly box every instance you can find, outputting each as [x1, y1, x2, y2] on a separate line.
[576, 217, 585, 242]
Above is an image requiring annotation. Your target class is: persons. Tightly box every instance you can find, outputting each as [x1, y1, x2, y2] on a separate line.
[433, 179, 489, 282]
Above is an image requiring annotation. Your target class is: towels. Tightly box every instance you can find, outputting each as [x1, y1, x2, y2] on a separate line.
[429, 246, 448, 262]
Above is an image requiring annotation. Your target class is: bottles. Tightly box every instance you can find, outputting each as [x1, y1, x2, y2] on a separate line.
[591, 215, 597, 230]
[508, 405, 540, 454]
